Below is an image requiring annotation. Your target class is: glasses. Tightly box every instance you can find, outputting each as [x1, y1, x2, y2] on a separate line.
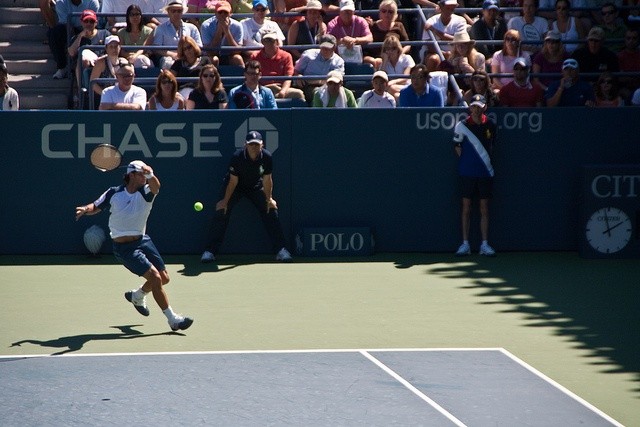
[516, 68, 527, 71]
[381, 9, 395, 13]
[202, 74, 215, 78]
[473, 78, 484, 81]
[411, 75, 423, 80]
[557, 7, 568, 10]
[506, 38, 517, 41]
[130, 13, 140, 16]
[601, 80, 613, 83]
[218, 11, 228, 14]
[162, 80, 172, 84]
[84, 19, 95, 23]
[246, 73, 260, 76]
[602, 10, 615, 15]
[590, 40, 598, 42]
[627, 36, 636, 40]
[342, 12, 353, 14]
[171, 9, 183, 13]
[327, 83, 338, 87]
[117, 73, 133, 77]
[183, 45, 192, 51]
[215, 1, 231, 12]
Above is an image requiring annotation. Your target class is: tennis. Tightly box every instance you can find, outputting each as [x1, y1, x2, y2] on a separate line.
[195, 202, 203, 211]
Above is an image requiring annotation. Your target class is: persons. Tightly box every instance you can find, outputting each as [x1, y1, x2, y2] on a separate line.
[75, 159, 193, 331]
[143, 1, 189, 26]
[631, 87, 640, 107]
[587, 1, 615, 26]
[614, 0, 640, 22]
[312, 71, 358, 107]
[365, 0, 411, 54]
[231, 0, 271, 22]
[464, 69, 501, 107]
[490, 29, 533, 94]
[187, 0, 232, 32]
[539, 0, 588, 19]
[150, 1, 204, 70]
[357, 71, 397, 108]
[293, 34, 345, 108]
[354, 0, 406, 25]
[377, 34, 417, 101]
[498, 61, 546, 108]
[319, 1, 341, 25]
[572, 26, 620, 82]
[169, 36, 213, 101]
[398, 64, 444, 107]
[419, 0, 467, 61]
[468, 0, 507, 60]
[271, 0, 308, 32]
[116, 3, 156, 69]
[149, 69, 186, 111]
[200, 130, 294, 263]
[287, 0, 327, 60]
[0, 64, 20, 113]
[89, 35, 129, 108]
[594, 72, 625, 107]
[97, 66, 147, 110]
[549, 0, 586, 63]
[544, 59, 597, 108]
[617, 28, 640, 97]
[586, 3, 630, 53]
[247, 32, 305, 100]
[412, 0, 476, 40]
[200, 1, 245, 67]
[240, 0, 286, 57]
[185, 64, 229, 110]
[326, 0, 373, 49]
[228, 60, 279, 109]
[499, 0, 523, 23]
[100, 0, 144, 35]
[447, 31, 486, 92]
[577, 14, 594, 35]
[453, 94, 501, 257]
[507, 0, 549, 61]
[47, 0, 101, 80]
[68, 10, 112, 95]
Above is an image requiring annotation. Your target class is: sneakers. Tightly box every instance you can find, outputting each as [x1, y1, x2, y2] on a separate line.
[276, 248, 293, 262]
[125, 290, 149, 316]
[456, 244, 470, 255]
[201, 251, 216, 263]
[480, 244, 496, 257]
[168, 315, 193, 331]
[53, 69, 67, 80]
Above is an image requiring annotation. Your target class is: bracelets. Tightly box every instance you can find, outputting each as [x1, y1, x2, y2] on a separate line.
[533, 30, 572, 93]
[143, 172, 155, 179]
[558, 87, 563, 91]
[145, 172, 155, 179]
[319, 80, 322, 85]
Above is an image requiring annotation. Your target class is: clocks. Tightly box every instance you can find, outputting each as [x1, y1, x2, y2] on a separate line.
[586, 207, 632, 255]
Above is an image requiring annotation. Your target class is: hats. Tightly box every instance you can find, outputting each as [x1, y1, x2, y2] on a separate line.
[562, 58, 578, 70]
[482, 0, 499, 12]
[512, 58, 527, 69]
[253, 0, 268, 8]
[470, 94, 487, 108]
[105, 35, 120, 44]
[247, 131, 263, 145]
[340, 0, 355, 11]
[439, 0, 459, 7]
[372, 71, 388, 83]
[586, 26, 606, 40]
[263, 33, 277, 40]
[448, 32, 475, 45]
[544, 30, 561, 40]
[127, 160, 147, 175]
[327, 70, 343, 84]
[297, 0, 327, 15]
[81, 10, 96, 21]
[159, 0, 189, 11]
[320, 34, 336, 48]
